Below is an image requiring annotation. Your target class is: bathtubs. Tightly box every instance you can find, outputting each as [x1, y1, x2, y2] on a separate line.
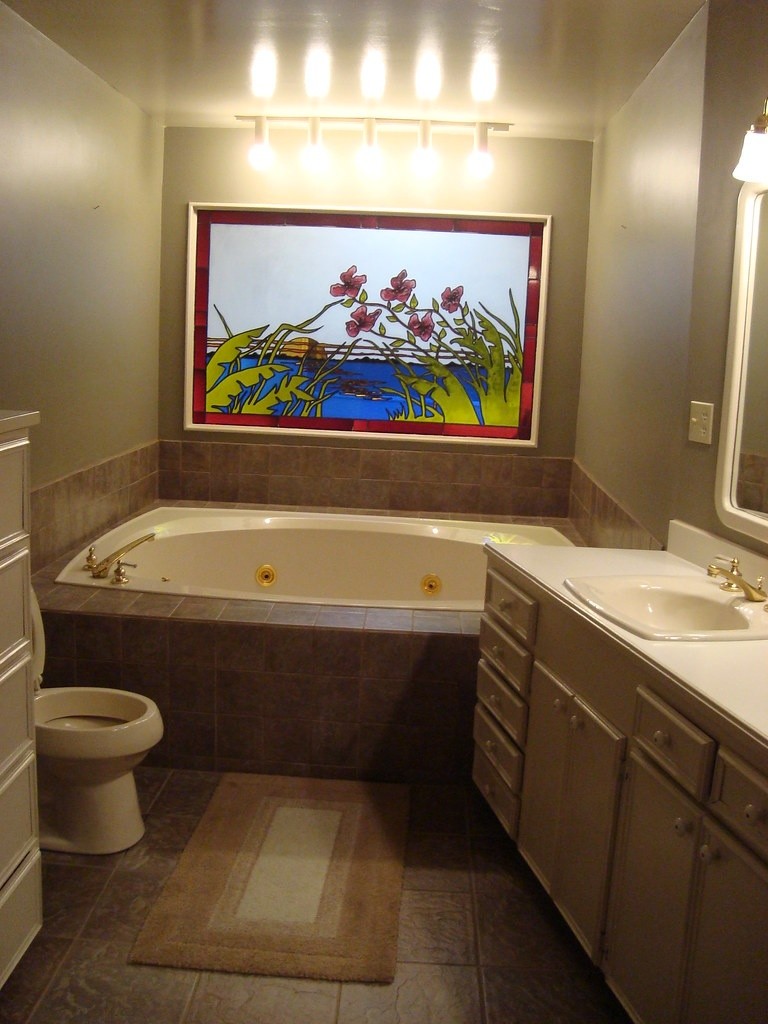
[30, 499, 589, 787]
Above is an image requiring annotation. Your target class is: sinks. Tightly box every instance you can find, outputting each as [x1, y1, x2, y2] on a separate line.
[563, 575, 768, 642]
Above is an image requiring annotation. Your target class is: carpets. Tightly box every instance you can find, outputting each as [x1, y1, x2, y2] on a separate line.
[129, 771, 411, 985]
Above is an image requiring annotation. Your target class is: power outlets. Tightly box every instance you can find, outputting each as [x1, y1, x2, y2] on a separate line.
[688, 400, 714, 445]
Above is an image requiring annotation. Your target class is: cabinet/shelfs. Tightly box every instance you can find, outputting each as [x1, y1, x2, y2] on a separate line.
[0, 403, 43, 991]
[518, 591, 628, 980]
[471, 542, 541, 861]
[601, 658, 768, 1024]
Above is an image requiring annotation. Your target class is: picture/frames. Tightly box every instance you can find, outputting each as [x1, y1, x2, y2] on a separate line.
[184, 202, 553, 449]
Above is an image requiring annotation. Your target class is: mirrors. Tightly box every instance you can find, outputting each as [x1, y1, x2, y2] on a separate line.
[714, 183, 768, 544]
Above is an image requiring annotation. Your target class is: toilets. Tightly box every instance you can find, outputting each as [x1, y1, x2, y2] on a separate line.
[30, 583, 164, 854]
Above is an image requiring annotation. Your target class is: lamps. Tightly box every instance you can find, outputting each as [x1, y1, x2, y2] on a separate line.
[235, 116, 516, 184]
[732, 96, 768, 185]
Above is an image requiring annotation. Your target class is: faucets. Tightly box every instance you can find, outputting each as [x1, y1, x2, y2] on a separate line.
[92, 533, 156, 578]
[707, 564, 768, 601]
